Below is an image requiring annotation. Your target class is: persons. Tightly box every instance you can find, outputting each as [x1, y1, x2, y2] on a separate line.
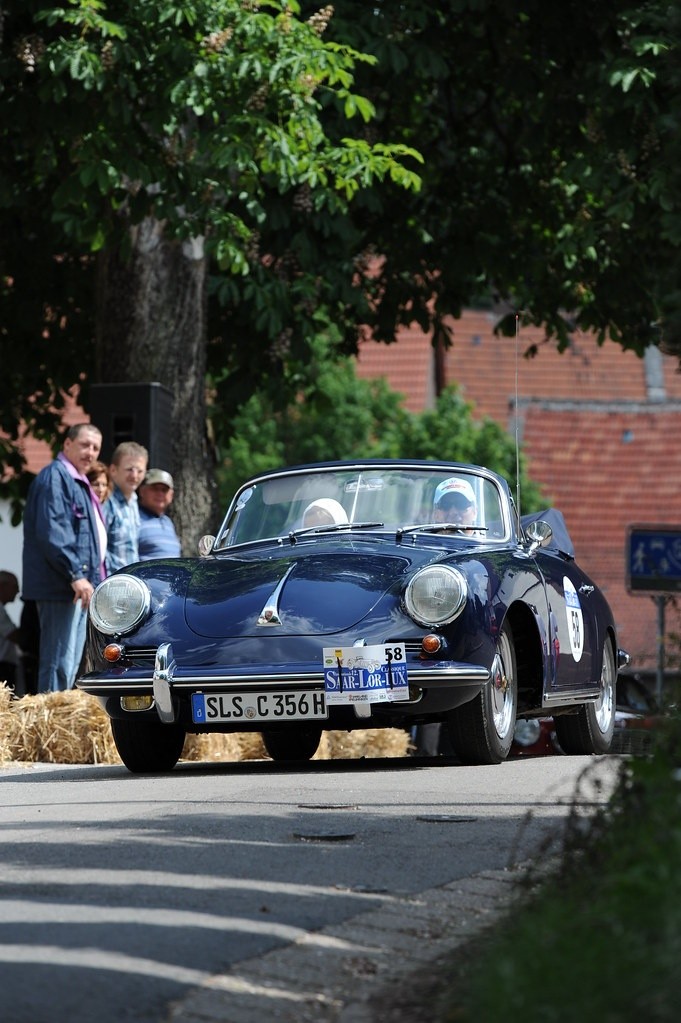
[135, 468, 182, 561]
[0, 569, 25, 694]
[299, 498, 350, 534]
[85, 461, 109, 581]
[99, 440, 149, 576]
[428, 477, 483, 539]
[19, 424, 103, 694]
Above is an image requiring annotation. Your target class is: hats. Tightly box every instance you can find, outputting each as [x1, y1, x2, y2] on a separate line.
[145, 468, 173, 489]
[433, 477, 477, 508]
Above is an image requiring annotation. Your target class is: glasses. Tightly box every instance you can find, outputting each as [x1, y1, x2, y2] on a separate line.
[435, 501, 472, 512]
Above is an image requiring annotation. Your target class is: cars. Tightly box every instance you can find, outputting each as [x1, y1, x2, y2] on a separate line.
[509, 673, 661, 757]
[107, 460, 620, 766]
